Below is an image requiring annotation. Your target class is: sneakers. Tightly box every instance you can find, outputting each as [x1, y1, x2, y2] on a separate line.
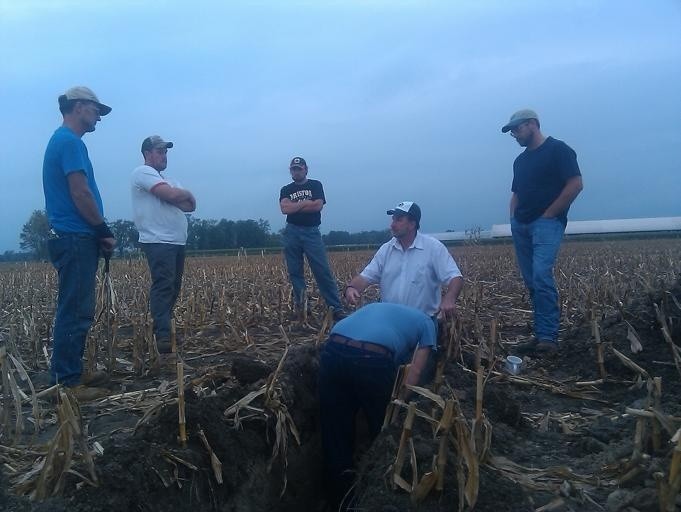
[285, 309, 307, 322]
[533, 340, 559, 359]
[516, 337, 537, 352]
[153, 336, 173, 353]
[329, 309, 347, 323]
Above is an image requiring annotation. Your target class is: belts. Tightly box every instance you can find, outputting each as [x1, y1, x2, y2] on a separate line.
[328, 334, 391, 357]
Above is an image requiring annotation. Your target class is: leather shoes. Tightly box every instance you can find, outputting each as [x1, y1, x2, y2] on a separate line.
[51, 384, 110, 401]
[81, 369, 108, 384]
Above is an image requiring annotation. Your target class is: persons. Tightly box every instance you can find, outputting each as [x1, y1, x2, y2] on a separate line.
[345, 201, 463, 340]
[131, 137, 196, 353]
[280, 158, 345, 323]
[316, 303, 436, 507]
[501, 111, 584, 351]
[44, 86, 118, 388]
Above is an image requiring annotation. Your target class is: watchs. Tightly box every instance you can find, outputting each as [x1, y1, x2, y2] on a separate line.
[346, 285, 354, 289]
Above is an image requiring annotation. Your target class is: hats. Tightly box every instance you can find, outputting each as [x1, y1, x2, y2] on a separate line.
[58, 85, 112, 117]
[141, 135, 173, 152]
[386, 201, 421, 219]
[289, 157, 306, 171]
